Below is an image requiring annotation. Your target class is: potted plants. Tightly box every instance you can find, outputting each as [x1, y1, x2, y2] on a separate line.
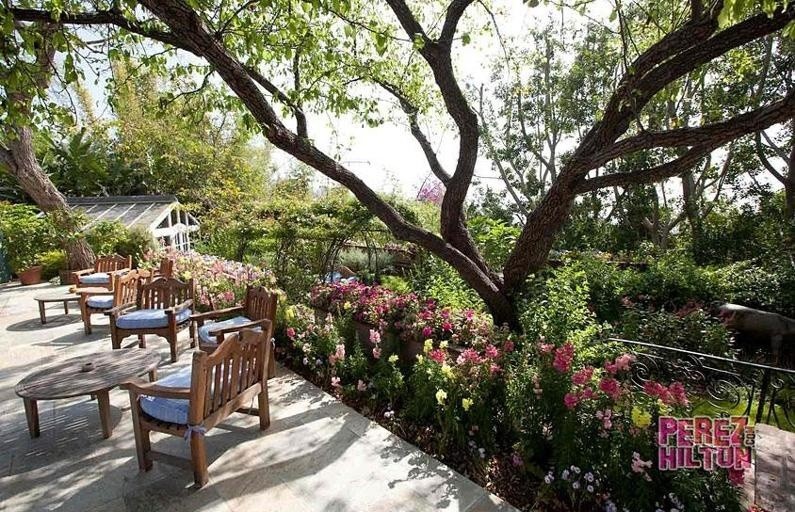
[0, 201, 96, 285]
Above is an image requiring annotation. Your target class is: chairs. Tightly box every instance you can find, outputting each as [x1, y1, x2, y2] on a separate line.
[104, 278, 194, 362]
[190, 283, 278, 378]
[76, 268, 154, 334]
[118, 319, 272, 487]
[153, 257, 172, 278]
[72, 254, 131, 305]
